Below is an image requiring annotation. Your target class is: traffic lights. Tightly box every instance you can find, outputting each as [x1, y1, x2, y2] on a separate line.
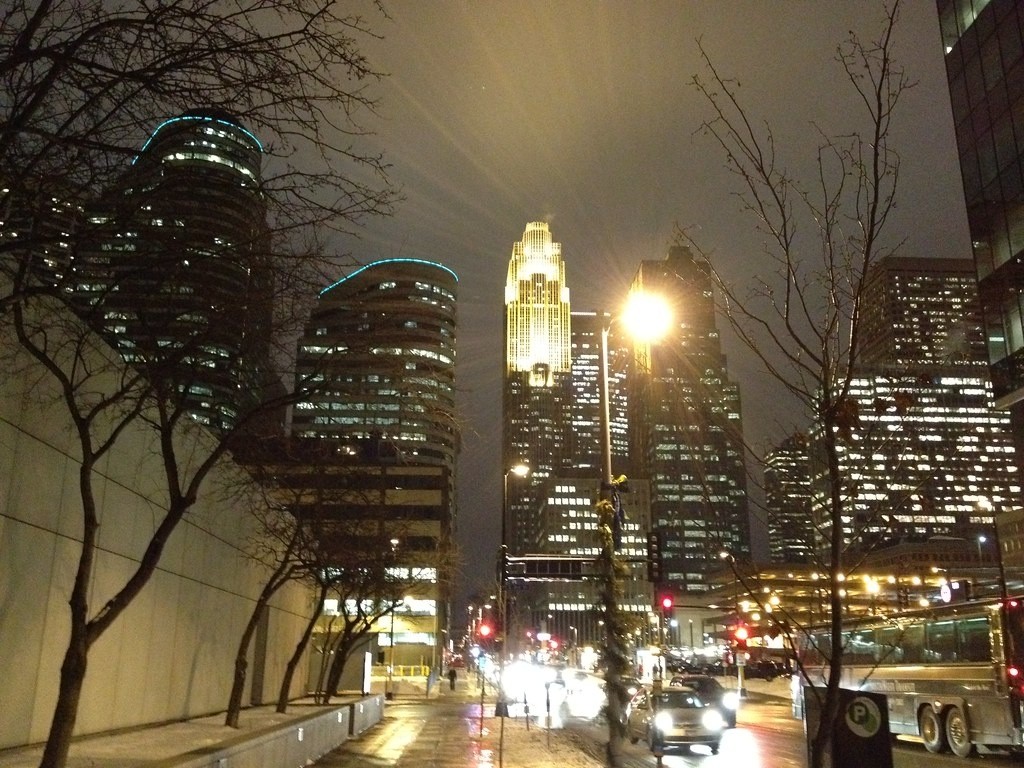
[736, 628, 746, 647]
[480, 624, 492, 637]
[663, 597, 673, 609]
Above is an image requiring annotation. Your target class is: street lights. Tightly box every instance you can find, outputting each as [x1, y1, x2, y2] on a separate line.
[386, 539, 399, 700]
[498, 465, 529, 767]
[595, 301, 664, 767]
[978, 501, 1006, 598]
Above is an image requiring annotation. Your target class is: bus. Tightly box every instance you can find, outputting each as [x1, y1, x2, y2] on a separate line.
[792, 596, 1024, 757]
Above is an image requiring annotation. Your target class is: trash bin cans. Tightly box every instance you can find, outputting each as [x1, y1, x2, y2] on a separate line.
[802, 684, 893, 767]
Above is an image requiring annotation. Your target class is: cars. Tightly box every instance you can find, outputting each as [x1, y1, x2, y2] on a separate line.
[622, 686, 723, 750]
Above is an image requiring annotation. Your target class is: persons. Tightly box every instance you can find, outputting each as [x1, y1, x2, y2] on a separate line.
[448, 668, 457, 691]
